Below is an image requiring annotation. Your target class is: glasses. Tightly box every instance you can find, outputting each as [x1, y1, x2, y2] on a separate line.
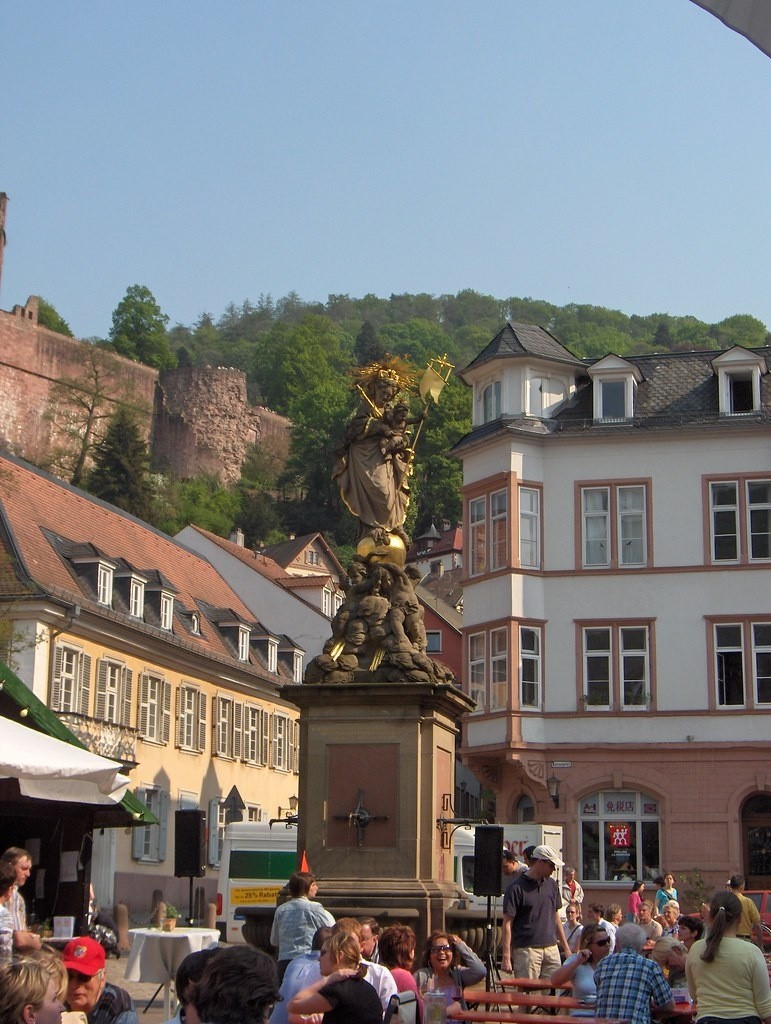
[591, 936, 610, 947]
[319, 950, 331, 957]
[363, 934, 376, 944]
[430, 945, 454, 955]
[65, 972, 103, 984]
[676, 926, 689, 931]
[540, 858, 556, 870]
[567, 911, 577, 915]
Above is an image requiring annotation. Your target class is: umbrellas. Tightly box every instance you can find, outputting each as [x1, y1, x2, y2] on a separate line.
[1, 718, 132, 807]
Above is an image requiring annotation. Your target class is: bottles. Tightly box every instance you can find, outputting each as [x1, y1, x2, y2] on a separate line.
[421, 990, 448, 1024]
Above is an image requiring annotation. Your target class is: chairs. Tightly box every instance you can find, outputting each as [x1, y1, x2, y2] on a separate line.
[382, 990, 420, 1024]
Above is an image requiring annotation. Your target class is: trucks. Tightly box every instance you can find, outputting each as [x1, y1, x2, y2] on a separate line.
[213, 822, 516, 965]
[467, 819, 564, 903]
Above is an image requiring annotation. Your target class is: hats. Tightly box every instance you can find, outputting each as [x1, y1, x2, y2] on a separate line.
[61, 936, 107, 978]
[531, 845, 566, 867]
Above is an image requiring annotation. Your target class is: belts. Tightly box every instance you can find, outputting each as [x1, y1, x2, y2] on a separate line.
[736, 934, 751, 939]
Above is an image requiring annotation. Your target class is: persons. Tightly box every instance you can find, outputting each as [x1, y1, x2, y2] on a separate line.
[412, 930, 487, 1024]
[163, 945, 280, 1024]
[0, 847, 42, 963]
[90, 905, 121, 960]
[378, 926, 463, 1024]
[0, 950, 67, 1024]
[270, 871, 337, 989]
[269, 917, 398, 1024]
[61, 936, 138, 1024]
[500, 845, 573, 1016]
[330, 363, 428, 544]
[552, 867, 771, 1024]
[324, 551, 428, 655]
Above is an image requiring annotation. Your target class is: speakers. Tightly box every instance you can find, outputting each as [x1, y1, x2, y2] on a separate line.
[175, 809, 207, 878]
[473, 826, 504, 897]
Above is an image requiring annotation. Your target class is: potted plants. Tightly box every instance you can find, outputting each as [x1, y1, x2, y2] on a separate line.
[37, 918, 54, 938]
[161, 902, 181, 929]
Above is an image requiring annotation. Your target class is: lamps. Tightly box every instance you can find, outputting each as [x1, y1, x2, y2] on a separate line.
[278, 794, 298, 818]
[547, 772, 560, 808]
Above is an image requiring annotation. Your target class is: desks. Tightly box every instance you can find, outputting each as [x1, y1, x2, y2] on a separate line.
[41, 935, 78, 944]
[125, 926, 222, 1024]
[447, 976, 699, 1024]
[614, 868, 638, 882]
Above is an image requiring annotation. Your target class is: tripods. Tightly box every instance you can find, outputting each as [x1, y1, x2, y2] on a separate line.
[478, 896, 513, 1024]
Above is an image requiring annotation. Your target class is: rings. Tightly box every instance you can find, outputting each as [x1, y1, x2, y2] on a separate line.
[454, 939, 456, 941]
